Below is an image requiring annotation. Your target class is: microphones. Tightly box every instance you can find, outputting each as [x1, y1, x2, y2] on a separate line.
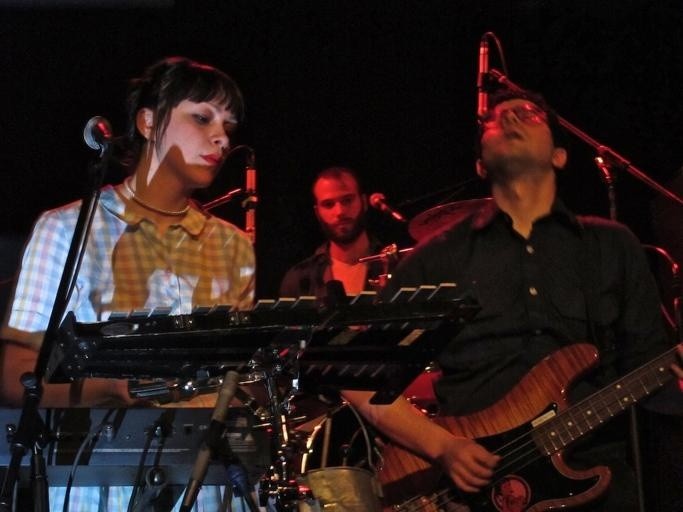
[242, 165, 257, 244]
[470, 38, 494, 127]
[368, 192, 405, 228]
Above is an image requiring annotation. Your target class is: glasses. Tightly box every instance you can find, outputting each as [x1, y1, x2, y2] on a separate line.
[479, 106, 544, 123]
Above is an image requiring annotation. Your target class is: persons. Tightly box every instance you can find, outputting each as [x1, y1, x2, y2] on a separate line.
[3, 57, 256, 512]
[297, 97, 683, 512]
[279, 167, 417, 470]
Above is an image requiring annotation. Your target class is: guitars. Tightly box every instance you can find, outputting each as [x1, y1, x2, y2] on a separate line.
[374, 343, 683, 510]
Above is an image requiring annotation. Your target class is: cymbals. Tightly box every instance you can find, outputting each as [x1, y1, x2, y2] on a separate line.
[408, 197, 497, 242]
